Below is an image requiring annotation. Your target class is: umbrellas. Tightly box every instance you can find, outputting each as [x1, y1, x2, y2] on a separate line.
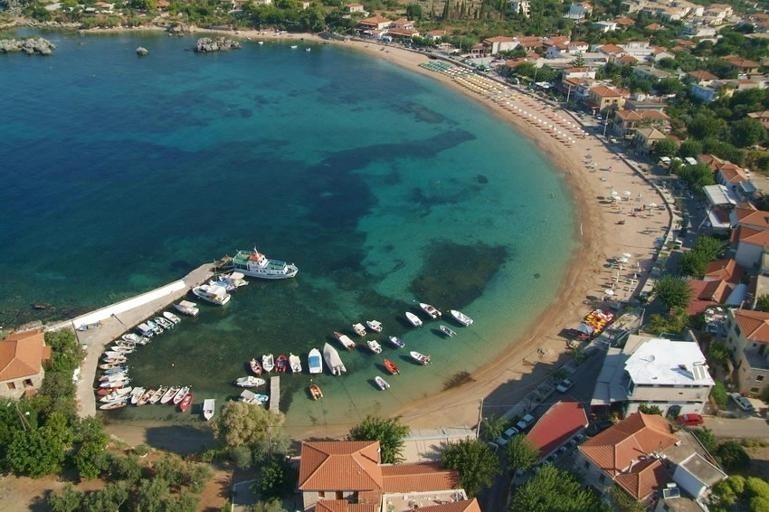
[647, 203, 657, 214]
[603, 252, 643, 300]
[610, 190, 631, 205]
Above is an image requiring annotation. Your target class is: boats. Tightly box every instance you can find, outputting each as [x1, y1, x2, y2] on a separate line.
[333, 330, 356, 352]
[450, 310, 473, 327]
[352, 320, 366, 337]
[389, 335, 406, 349]
[308, 347, 323, 374]
[234, 351, 303, 407]
[366, 320, 383, 333]
[406, 312, 423, 327]
[410, 351, 431, 365]
[366, 340, 382, 355]
[323, 342, 347, 376]
[419, 302, 442, 319]
[375, 376, 391, 391]
[309, 383, 324, 401]
[203, 398, 215, 421]
[384, 358, 400, 376]
[172, 299, 199, 316]
[440, 324, 457, 338]
[231, 249, 299, 279]
[96, 311, 191, 412]
[193, 270, 248, 305]
